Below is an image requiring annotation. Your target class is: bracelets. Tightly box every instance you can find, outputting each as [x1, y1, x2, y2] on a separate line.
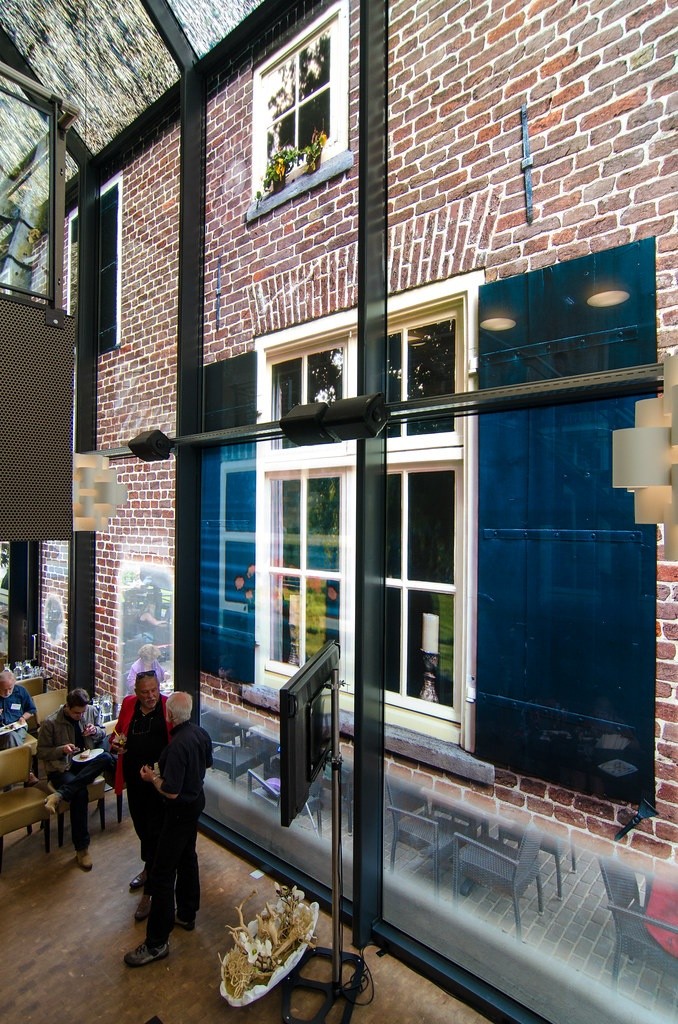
[153, 774, 160, 781]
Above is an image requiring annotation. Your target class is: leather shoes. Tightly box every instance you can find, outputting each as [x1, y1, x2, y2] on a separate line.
[135, 895, 153, 919]
[130, 871, 148, 887]
[26, 772, 39, 787]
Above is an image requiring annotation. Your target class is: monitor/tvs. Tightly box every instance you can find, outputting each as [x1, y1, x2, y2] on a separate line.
[278, 640, 340, 827]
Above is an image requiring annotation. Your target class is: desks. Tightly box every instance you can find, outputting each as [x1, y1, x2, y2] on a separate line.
[15, 675, 52, 697]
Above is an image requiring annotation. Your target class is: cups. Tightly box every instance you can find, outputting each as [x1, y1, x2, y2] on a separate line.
[92, 695, 121, 716]
[1, 659, 46, 683]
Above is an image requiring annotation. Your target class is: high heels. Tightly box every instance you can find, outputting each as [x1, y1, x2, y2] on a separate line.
[76, 846, 93, 869]
[44, 793, 62, 814]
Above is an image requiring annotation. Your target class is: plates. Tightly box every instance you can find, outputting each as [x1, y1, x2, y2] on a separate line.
[72, 748, 104, 762]
[0, 720, 27, 736]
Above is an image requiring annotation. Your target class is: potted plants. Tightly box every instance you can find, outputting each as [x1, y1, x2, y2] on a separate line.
[217, 883, 319, 1007]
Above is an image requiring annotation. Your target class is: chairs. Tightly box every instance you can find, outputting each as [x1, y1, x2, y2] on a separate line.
[240, 728, 281, 757]
[200, 711, 269, 787]
[102, 718, 127, 823]
[32, 689, 68, 726]
[596, 855, 678, 1000]
[452, 825, 545, 941]
[386, 781, 476, 898]
[37, 724, 106, 848]
[497, 823, 578, 902]
[0, 743, 51, 872]
[247, 756, 323, 835]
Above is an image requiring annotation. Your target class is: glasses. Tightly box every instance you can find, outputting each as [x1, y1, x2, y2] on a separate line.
[135, 670, 158, 687]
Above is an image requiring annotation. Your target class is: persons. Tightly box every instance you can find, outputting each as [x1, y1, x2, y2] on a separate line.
[123, 691, 215, 968]
[129, 601, 167, 640]
[40, 687, 126, 872]
[126, 643, 169, 695]
[108, 670, 183, 921]
[0, 671, 38, 748]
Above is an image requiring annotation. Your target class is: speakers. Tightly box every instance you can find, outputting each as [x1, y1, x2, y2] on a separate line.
[127, 430, 172, 463]
[324, 391, 389, 441]
[277, 402, 342, 446]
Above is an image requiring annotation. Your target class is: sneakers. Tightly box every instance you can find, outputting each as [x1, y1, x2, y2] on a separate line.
[125, 942, 170, 965]
[174, 909, 195, 931]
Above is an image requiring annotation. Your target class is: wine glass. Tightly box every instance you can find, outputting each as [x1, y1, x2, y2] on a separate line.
[115, 733, 127, 754]
